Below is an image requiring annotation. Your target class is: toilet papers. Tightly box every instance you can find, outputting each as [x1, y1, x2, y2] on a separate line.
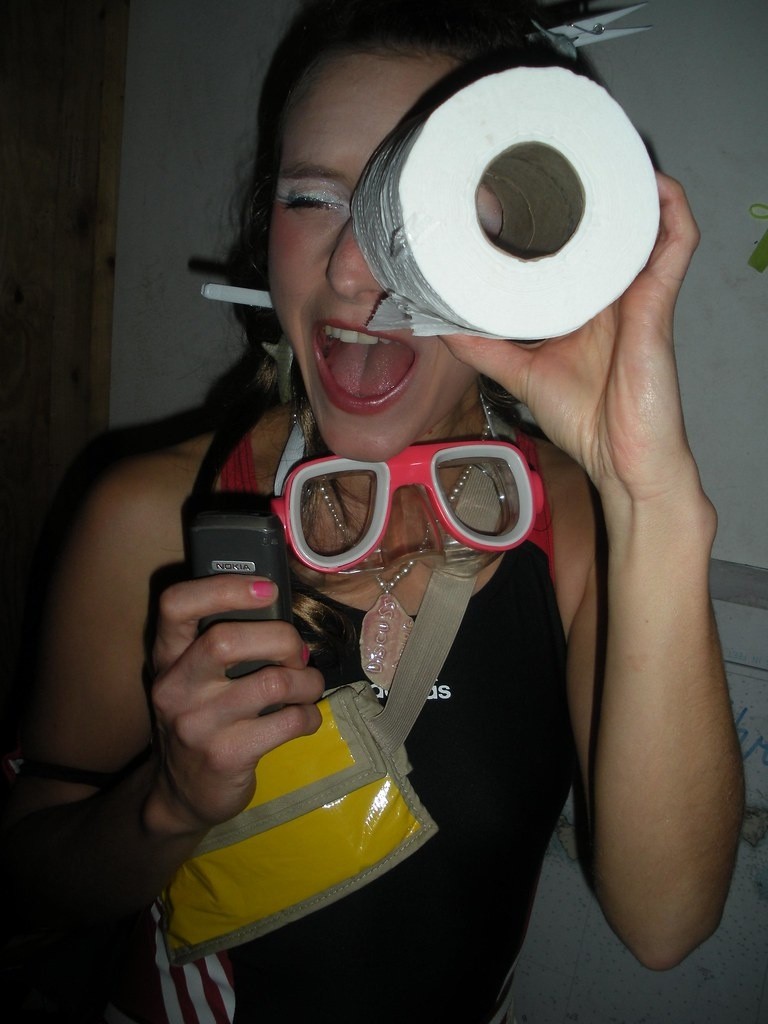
[348, 66, 661, 341]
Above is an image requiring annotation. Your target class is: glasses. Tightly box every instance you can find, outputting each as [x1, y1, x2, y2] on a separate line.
[269, 406, 546, 574]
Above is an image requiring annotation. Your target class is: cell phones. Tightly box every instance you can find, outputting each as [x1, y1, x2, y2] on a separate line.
[192, 514, 296, 716]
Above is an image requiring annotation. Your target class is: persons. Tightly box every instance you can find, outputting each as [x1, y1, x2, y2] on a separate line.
[0, 0, 746, 1024]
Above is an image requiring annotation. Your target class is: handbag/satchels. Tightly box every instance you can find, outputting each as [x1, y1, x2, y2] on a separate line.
[154, 679, 440, 964]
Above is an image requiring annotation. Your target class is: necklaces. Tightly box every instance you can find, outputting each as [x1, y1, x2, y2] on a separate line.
[289, 392, 492, 692]
